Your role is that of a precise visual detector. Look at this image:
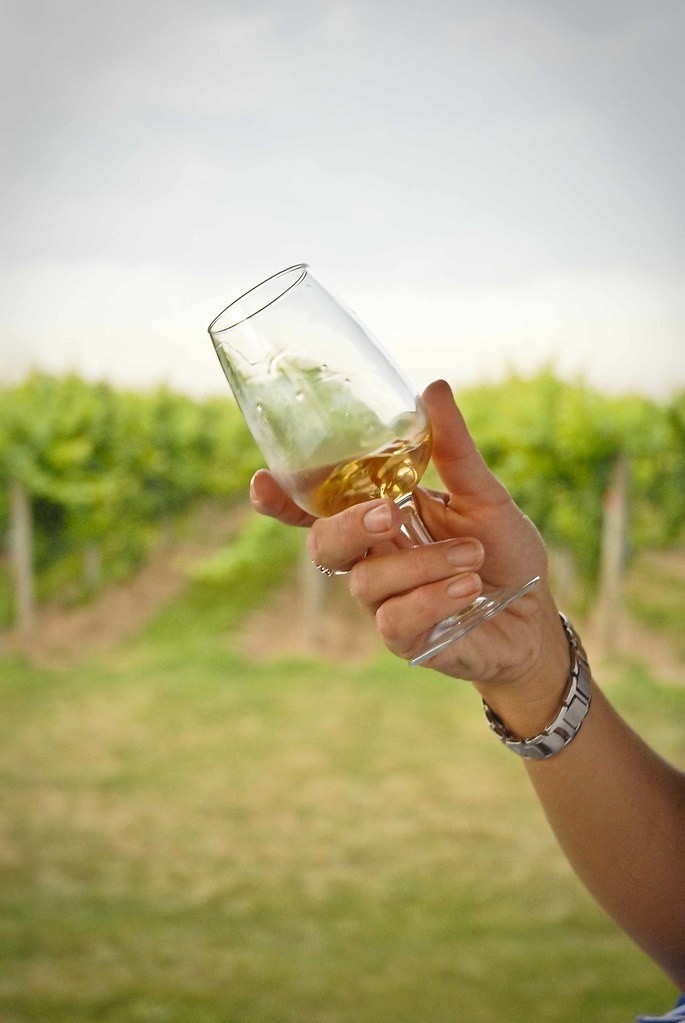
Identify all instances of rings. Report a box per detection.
[309,547,367,578]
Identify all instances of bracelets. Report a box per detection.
[481,609,593,762]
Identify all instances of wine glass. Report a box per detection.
[208,264,540,668]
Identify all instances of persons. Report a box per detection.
[249,377,685,1023]
